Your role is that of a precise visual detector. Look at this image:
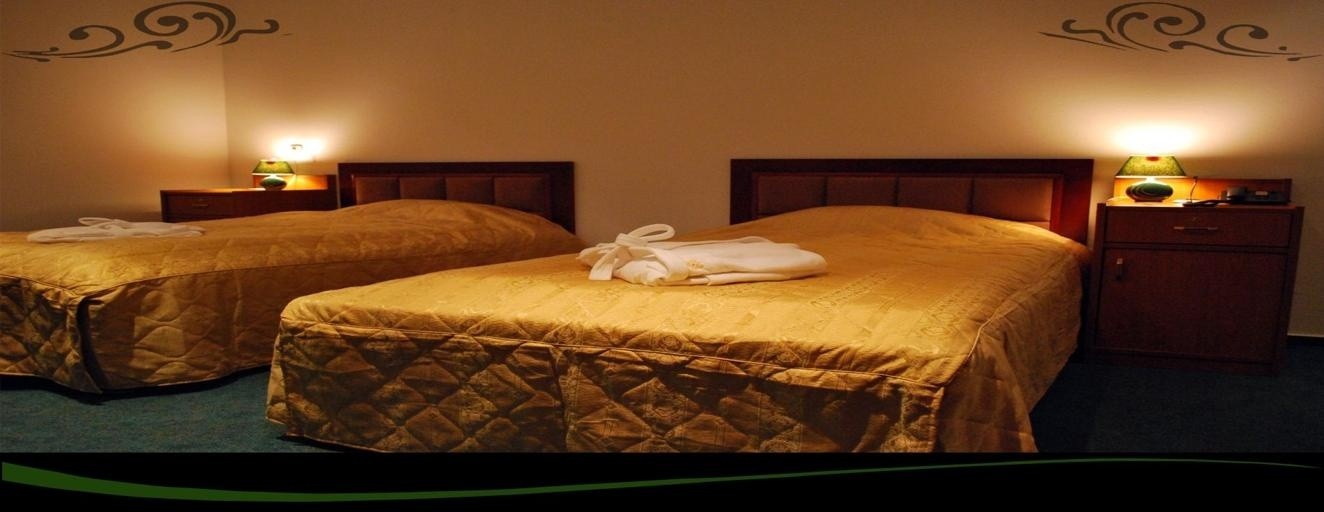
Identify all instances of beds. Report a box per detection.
[0,159,592,409]
[264,154,1095,456]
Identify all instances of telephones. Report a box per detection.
[1226,186,1287,203]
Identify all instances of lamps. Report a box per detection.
[1113,155,1187,202]
[251,159,295,190]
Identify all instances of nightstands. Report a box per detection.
[1082,173,1305,371]
[158,171,338,223]
